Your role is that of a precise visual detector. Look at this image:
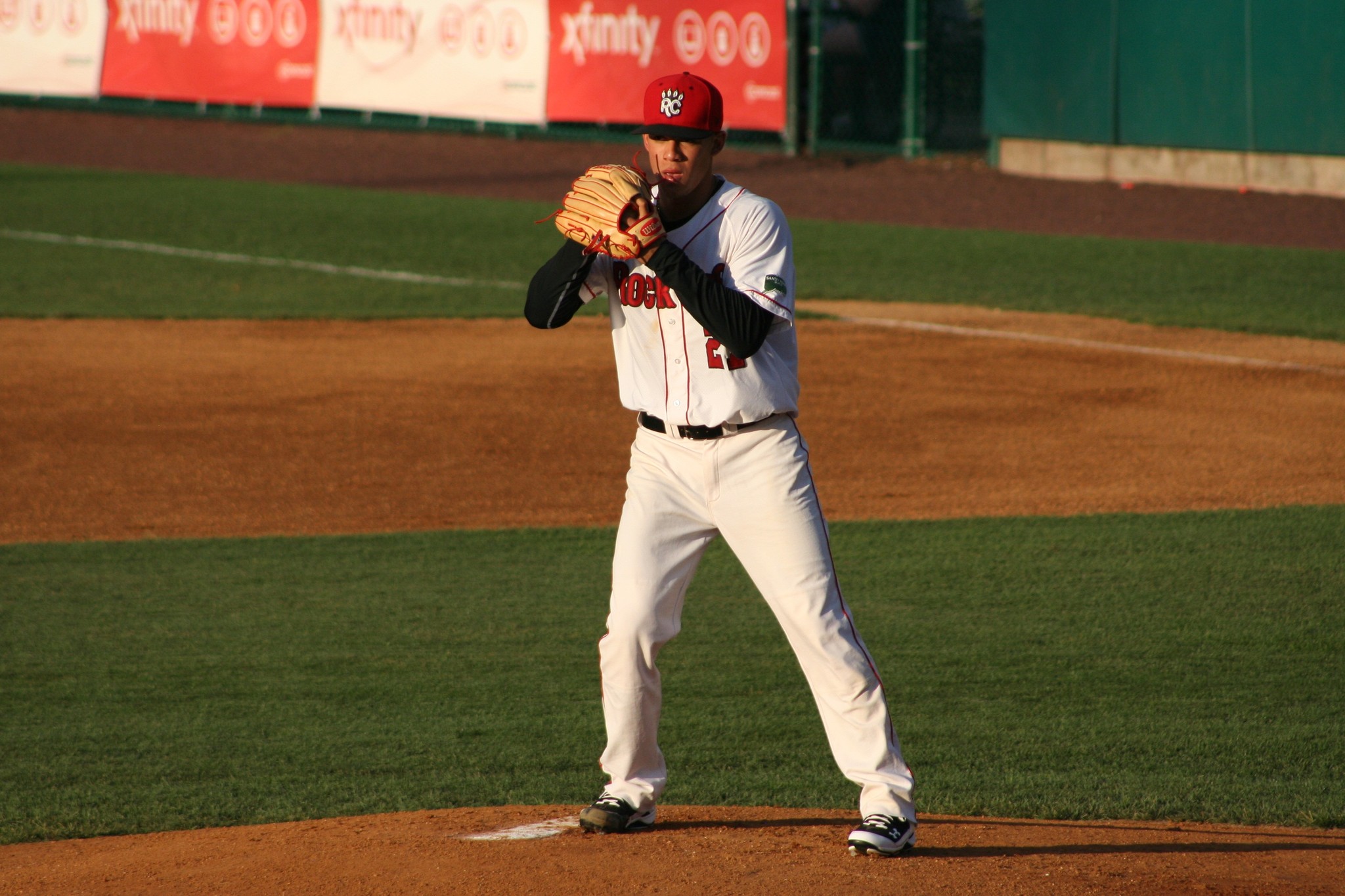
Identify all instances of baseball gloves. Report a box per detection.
[552,165,668,261]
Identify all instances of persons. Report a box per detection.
[519,71,919,860]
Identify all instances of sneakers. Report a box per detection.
[847,814,916,853]
[578,791,658,833]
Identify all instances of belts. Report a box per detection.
[640,411,774,440]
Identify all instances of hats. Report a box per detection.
[630,72,724,136]
